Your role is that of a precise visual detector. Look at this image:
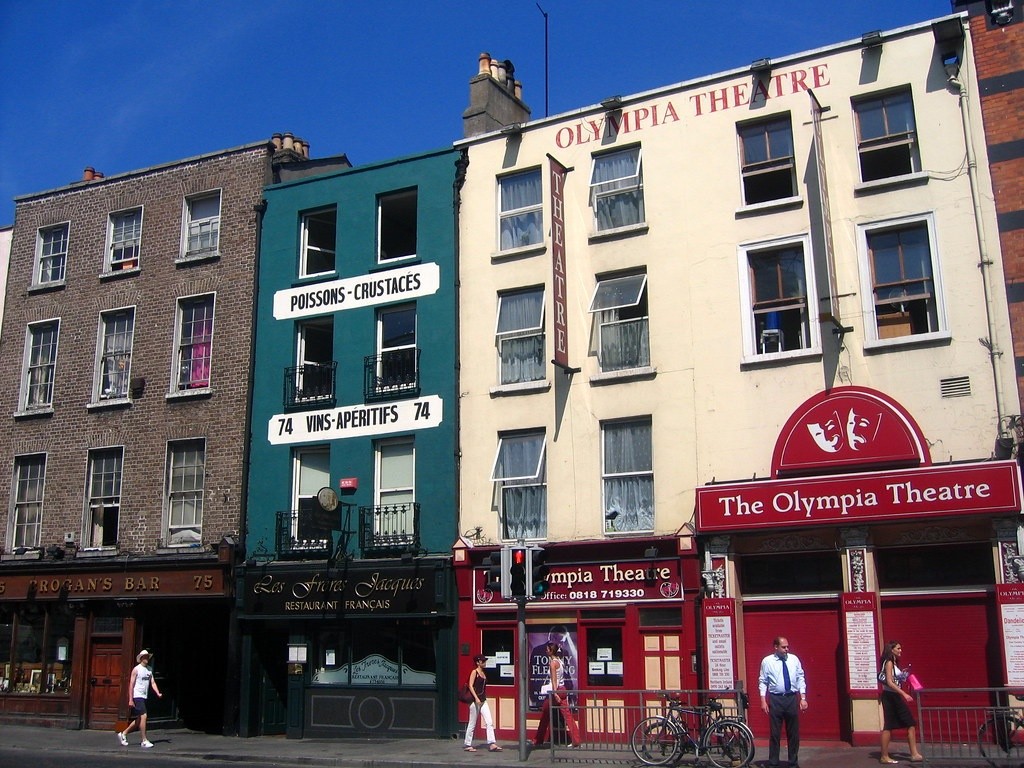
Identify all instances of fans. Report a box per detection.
[889,287,909,313]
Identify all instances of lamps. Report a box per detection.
[14,547,45,557]
[645,545,658,580]
[861,30,883,48]
[246,553,275,566]
[501,122,522,135]
[601,95,624,111]
[401,548,428,564]
[46,546,65,559]
[750,57,772,75]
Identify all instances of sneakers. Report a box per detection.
[118,732,129,746]
[140,741,153,748]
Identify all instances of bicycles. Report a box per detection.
[631,689,756,768]
[977,693,1024,768]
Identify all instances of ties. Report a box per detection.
[782,658,791,691]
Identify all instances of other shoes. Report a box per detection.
[880,758,899,764]
[912,756,929,762]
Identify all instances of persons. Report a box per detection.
[879,641,928,763]
[532,644,582,748]
[758,636,808,768]
[463,654,503,751]
[529,625,574,708]
[117,650,162,748]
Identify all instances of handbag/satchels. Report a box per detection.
[540,685,552,694]
[460,670,477,703]
[879,659,899,687]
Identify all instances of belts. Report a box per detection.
[775,693,795,696]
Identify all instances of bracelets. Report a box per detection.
[801,698,807,700]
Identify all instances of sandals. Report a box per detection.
[488,745,503,750]
[463,746,477,752]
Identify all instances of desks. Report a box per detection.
[877,311,914,338]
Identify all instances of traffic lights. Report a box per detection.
[529,548,551,599]
[508,547,530,599]
[489,548,506,599]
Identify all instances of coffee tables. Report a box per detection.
[762,329,782,353]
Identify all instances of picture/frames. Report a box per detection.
[29,669,50,692]
[4,662,10,680]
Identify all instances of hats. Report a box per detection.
[136,649,152,663]
[474,654,488,663]
